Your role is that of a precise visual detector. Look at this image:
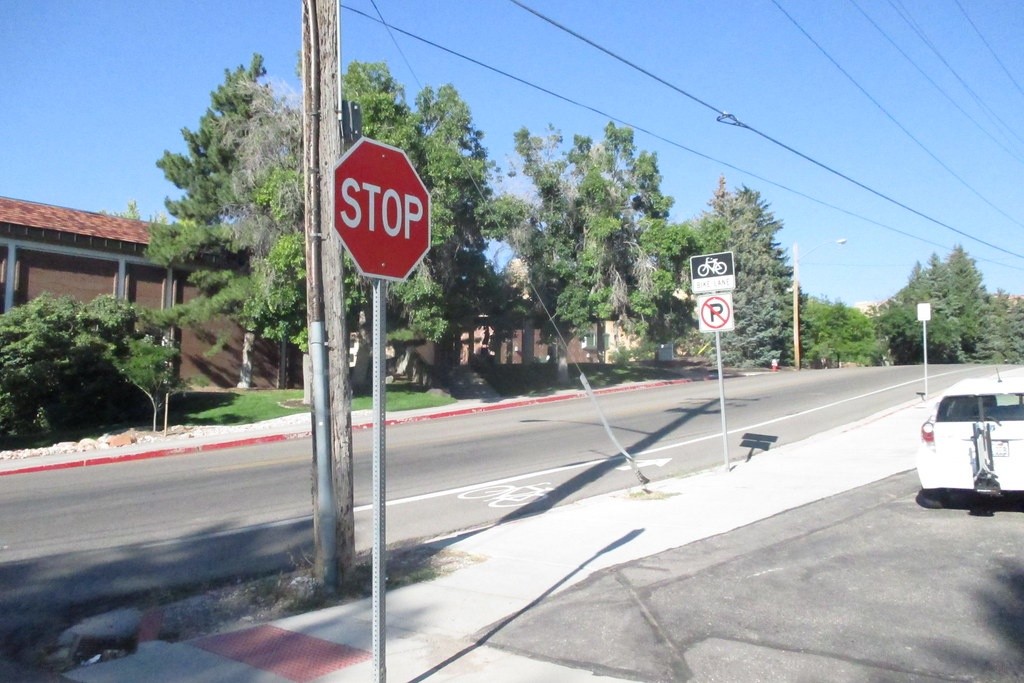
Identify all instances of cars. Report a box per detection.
[917,371,1024,495]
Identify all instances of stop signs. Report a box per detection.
[331,136,432,282]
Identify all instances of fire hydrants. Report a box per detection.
[771,358,779,372]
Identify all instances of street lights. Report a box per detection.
[793,237,846,372]
